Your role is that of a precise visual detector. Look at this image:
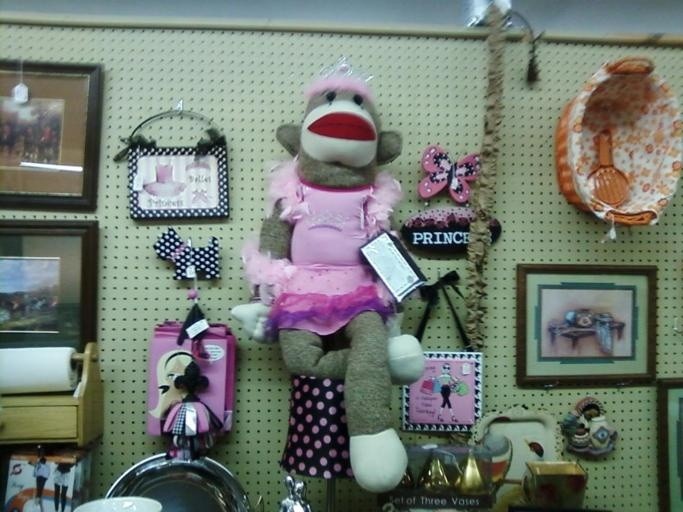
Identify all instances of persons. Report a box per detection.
[430,361,459,421]
[1,102,62,165]
[28,457,51,512]
[53,461,75,512]
[149,349,201,422]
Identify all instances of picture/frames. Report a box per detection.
[515,262,658,391]
[654,376,683,512]
[0,59,102,354]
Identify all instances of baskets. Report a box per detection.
[556,58,683,224]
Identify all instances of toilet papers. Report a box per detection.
[0,347,76,395]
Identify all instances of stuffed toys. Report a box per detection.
[230,68,426,493]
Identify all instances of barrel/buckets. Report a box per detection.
[106,455,250,510]
